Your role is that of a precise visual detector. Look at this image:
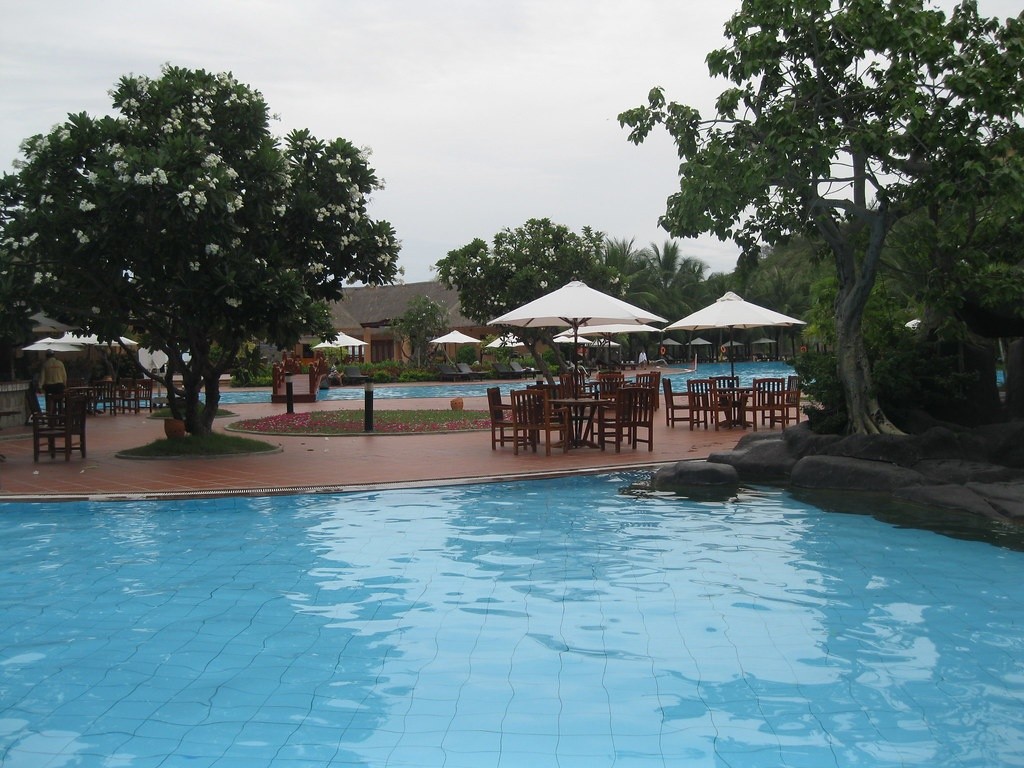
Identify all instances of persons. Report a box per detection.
[639,349,647,370]
[331,361,345,386]
[510,351,519,359]
[38,349,66,425]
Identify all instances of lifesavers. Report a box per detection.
[721,345,726,353]
[661,347,665,354]
[800,346,806,353]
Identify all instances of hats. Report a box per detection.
[45,349,54,355]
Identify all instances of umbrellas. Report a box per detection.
[685,337,712,345]
[752,337,776,353]
[313,332,368,359]
[722,341,744,347]
[485,332,531,348]
[23,326,138,359]
[551,323,665,371]
[663,292,806,388]
[429,330,481,353]
[486,281,667,398]
[656,338,683,346]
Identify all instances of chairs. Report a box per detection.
[345,366,368,385]
[597,358,639,371]
[492,362,540,380]
[438,363,487,382]
[487,371,661,456]
[663,376,801,432]
[25,378,154,463]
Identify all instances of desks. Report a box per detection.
[707,388,762,428]
[548,400,610,449]
[590,380,633,398]
